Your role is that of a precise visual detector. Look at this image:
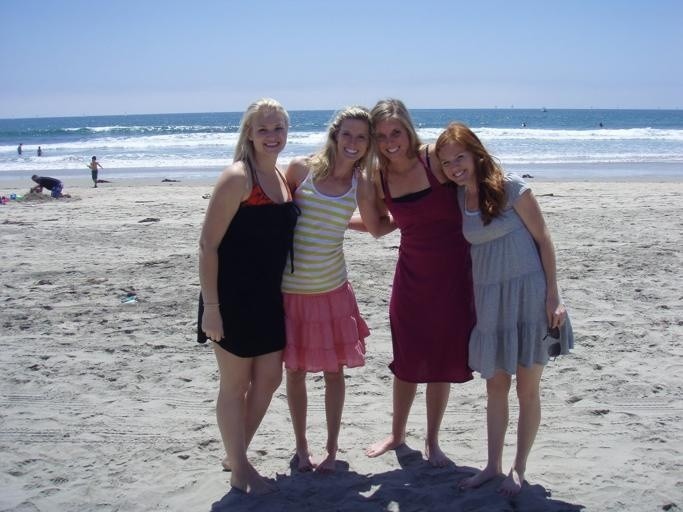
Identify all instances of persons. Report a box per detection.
[31,175,71,199]
[347,99,477,468]
[87,156,103,188]
[281,107,398,472]
[17,143,22,155]
[434,124,568,498]
[196,98,301,495]
[36,146,42,157]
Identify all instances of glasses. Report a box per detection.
[542,327,560,361]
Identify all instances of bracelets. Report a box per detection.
[203,304,219,307]
